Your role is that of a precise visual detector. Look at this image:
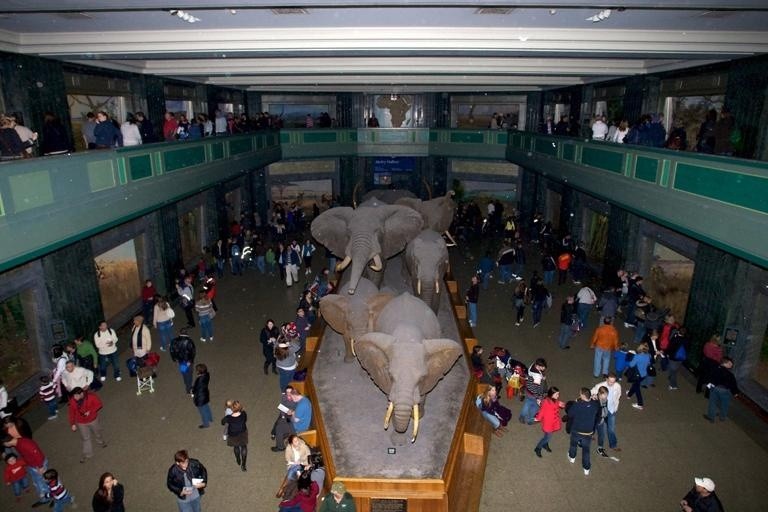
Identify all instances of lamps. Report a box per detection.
[166,7,201,25]
[585,8,612,24]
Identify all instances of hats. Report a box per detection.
[694,477,715,493]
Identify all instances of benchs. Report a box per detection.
[408,245,505,511]
[285,247,373,511]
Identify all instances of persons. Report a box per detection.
[541,109,758,162]
[304,111,332,130]
[1,111,39,161]
[368,113,380,128]
[42,112,71,156]
[81,110,282,151]
[490,112,519,129]
[0,190,740,512]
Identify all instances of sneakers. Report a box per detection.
[626,383,728,423]
[535,440,622,476]
[48,372,123,465]
[271,446,285,452]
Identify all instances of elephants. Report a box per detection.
[319,277,395,363]
[401,228,450,317]
[354,290,463,443]
[393,190,458,247]
[311,196,424,295]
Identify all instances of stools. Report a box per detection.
[135,366,153,395]
